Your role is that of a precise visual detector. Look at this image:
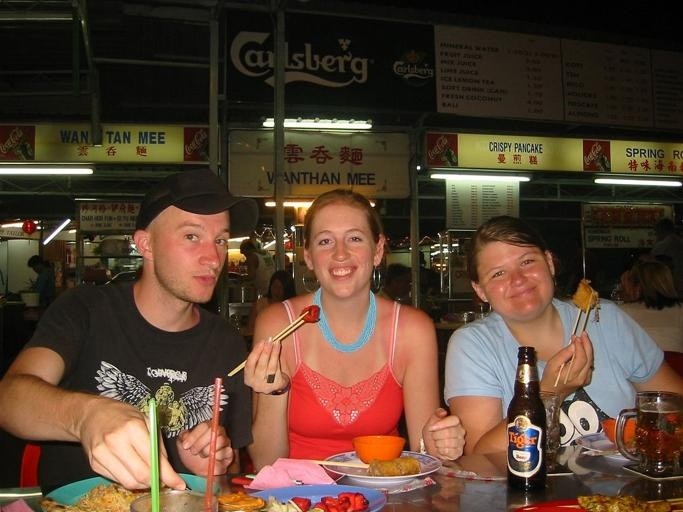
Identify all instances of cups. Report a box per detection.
[538,391,561,472]
[614,390,683,476]
[129,489,218,511]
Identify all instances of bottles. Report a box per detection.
[507,347,547,491]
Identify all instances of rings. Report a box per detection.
[267,374,276,383]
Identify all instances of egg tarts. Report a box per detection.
[218,493,265,511]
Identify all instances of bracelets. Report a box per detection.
[263,373,291,395]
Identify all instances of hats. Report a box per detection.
[136,170,259,238]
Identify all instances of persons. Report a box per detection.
[2,170,254,491]
[28,254,60,304]
[238,241,276,295]
[442,216,681,454]
[650,219,679,264]
[243,189,466,480]
[618,259,681,311]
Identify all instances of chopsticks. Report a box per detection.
[552,290,594,388]
[226,308,311,379]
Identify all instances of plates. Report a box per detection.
[321,450,442,490]
[247,484,387,512]
[575,432,637,458]
[44,472,222,512]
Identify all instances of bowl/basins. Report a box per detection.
[351,435,406,464]
[600,417,636,445]
